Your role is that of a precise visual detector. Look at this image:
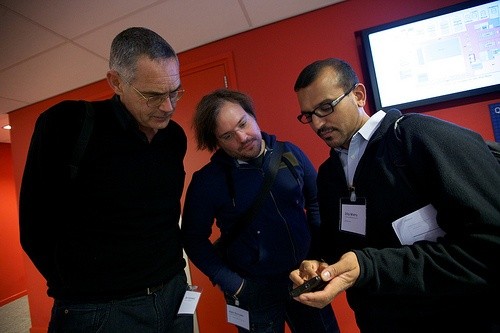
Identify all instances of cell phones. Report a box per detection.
[291,275,328,297]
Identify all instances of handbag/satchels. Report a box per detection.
[209,236,233,287]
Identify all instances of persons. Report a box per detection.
[290,58,500,333]
[19,27,194,333]
[181,89,341,333]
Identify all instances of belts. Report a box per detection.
[119,257,187,301]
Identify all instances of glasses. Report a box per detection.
[118,73,185,109]
[296,82,359,125]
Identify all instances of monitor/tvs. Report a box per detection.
[359,0,500,111]
